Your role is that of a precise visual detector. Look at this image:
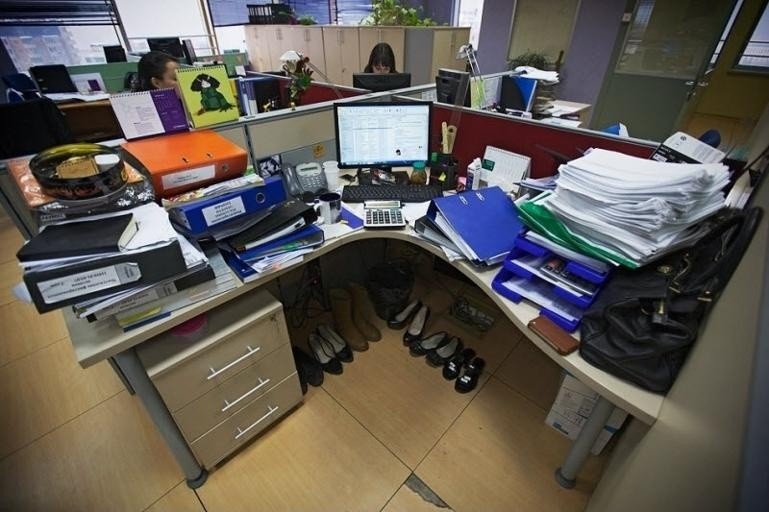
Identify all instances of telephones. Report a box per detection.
[281,161,329,197]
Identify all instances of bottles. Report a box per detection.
[323,161,340,189]
[411,163,427,186]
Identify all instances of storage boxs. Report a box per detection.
[544,368,630,456]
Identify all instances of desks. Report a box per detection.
[63,166,669,489]
[57,91,113,110]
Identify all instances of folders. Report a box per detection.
[120,128,248,202]
[169,176,288,234]
[226,197,317,254]
[510,75,539,114]
[413,186,530,267]
[235,224,324,264]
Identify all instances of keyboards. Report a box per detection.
[342,184,444,203]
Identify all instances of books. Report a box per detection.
[314,206,365,241]
[160,173,267,212]
[245,245,316,274]
[219,248,305,283]
[15,211,142,264]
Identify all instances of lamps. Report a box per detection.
[281,49,344,98]
[455,43,487,109]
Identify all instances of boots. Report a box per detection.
[329,282,380,352]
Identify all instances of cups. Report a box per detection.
[314,192,341,225]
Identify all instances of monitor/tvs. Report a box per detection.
[332,101,434,186]
[352,73,411,93]
[434,68,470,108]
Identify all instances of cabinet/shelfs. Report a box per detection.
[131,284,304,473]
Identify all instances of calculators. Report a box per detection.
[364,200,406,229]
[540,255,606,299]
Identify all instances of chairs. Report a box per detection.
[0,74,39,102]
[700,129,721,148]
[0,98,72,159]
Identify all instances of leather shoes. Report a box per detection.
[387,300,483,394]
[294,325,352,394]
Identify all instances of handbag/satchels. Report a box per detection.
[580,207,764,393]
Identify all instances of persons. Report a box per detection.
[362,42,402,75]
[130,51,181,99]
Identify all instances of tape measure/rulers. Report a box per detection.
[442,72,471,154]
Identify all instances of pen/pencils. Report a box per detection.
[404,220,419,235]
